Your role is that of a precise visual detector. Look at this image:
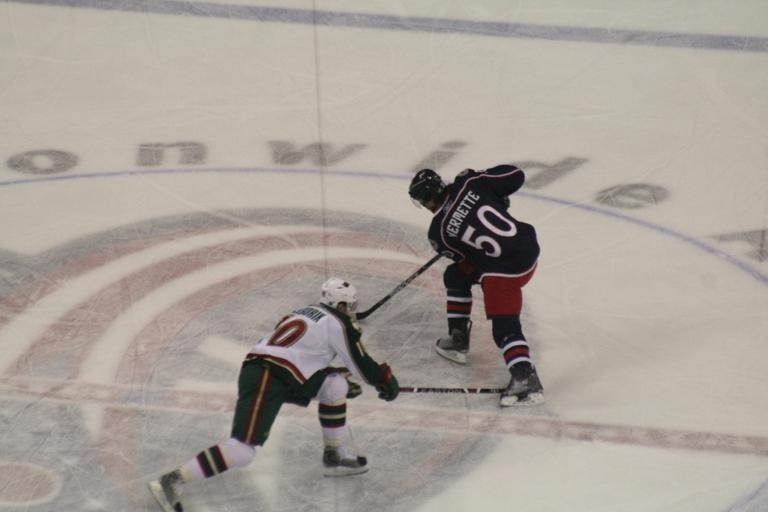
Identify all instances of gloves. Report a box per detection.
[375,361,400,401]
[338,366,362,399]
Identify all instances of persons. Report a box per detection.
[407,164,548,407]
[147,277,402,512]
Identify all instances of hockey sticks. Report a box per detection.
[355,253,442,318]
[399,374,516,393]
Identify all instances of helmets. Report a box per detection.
[317,277,358,313]
[408,168,449,214]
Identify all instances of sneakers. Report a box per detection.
[434,328,469,354]
[500,371,543,398]
[321,445,367,469]
[160,469,187,512]
